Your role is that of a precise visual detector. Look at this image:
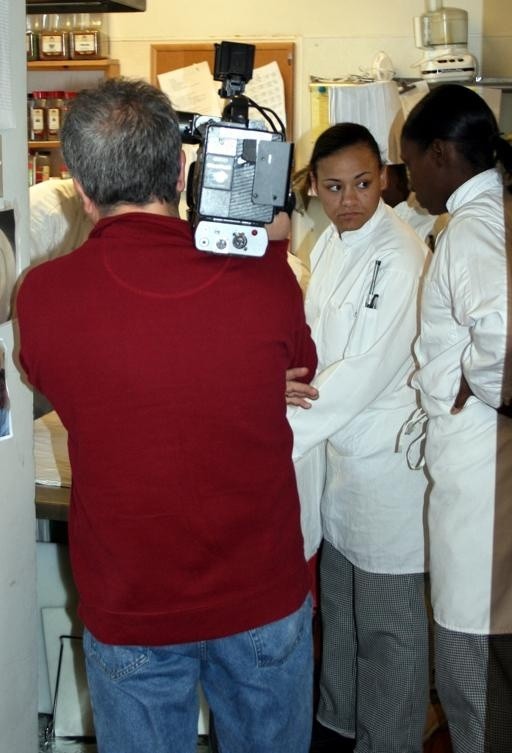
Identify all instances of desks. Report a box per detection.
[33,410,71,543]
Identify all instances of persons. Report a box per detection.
[388,76,510,751]
[9,72,326,751]
[281,119,428,751]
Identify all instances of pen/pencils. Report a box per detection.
[365,261,381,308]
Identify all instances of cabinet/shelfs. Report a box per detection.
[25,60,124,192]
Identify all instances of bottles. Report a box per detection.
[25,12,111,186]
